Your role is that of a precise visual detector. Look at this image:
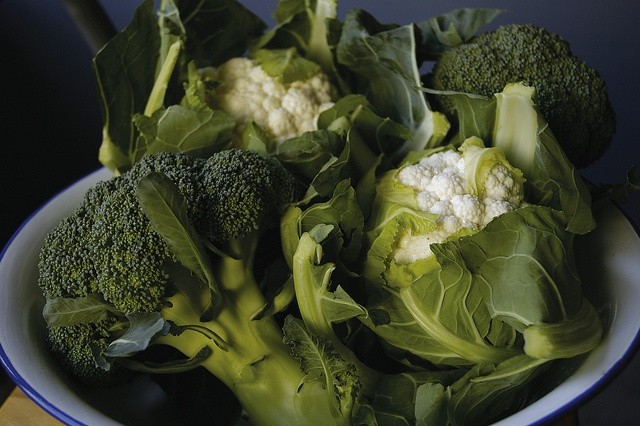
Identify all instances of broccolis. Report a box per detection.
[37,0,618,426]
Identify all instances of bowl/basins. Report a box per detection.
[2,167,638,425]
